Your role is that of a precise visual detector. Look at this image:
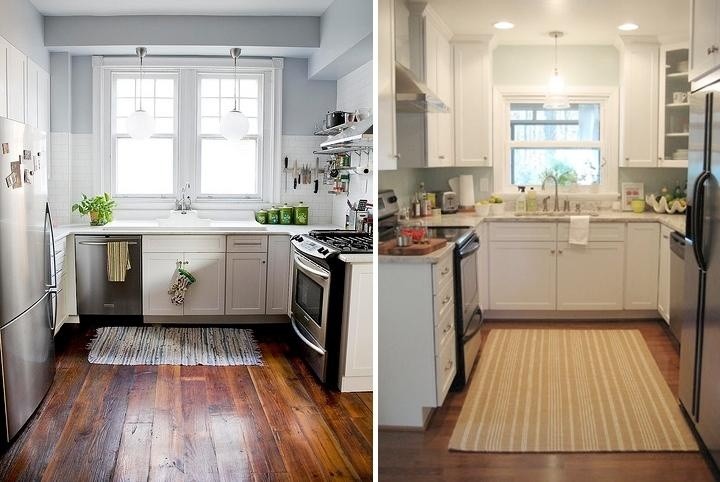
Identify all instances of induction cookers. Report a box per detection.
[378,227,475,248]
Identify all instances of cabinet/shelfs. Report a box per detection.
[658,224,677,326]
[485,220,626,311]
[379,239,458,433]
[227,235,269,316]
[626,222,662,311]
[52,237,68,335]
[687,0,720,82]
[617,34,657,167]
[269,234,292,315]
[377,1,403,171]
[311,122,359,173]
[418,5,457,168]
[142,236,228,316]
[453,40,494,168]
[658,42,691,168]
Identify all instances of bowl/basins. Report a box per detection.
[673,150,688,160]
[475,205,489,216]
[490,203,505,215]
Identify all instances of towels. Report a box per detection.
[568,215,593,246]
[105,240,132,284]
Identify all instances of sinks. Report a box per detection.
[513,211,594,221]
[158,218,211,228]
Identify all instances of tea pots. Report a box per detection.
[393,220,426,239]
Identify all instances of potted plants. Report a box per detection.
[68,190,118,227]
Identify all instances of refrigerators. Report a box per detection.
[2,116,60,447]
[676,92,720,474]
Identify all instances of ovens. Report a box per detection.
[453,233,484,390]
[288,251,345,387]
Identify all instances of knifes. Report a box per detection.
[292,156,319,194]
[285,156,289,193]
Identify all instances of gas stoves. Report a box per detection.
[289,231,373,268]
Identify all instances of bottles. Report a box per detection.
[256,201,309,225]
[674,179,687,198]
[411,183,432,217]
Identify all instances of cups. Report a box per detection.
[631,199,646,213]
[673,92,687,103]
[397,235,412,247]
[344,113,355,124]
[397,207,410,223]
[356,107,370,124]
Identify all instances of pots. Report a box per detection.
[326,111,353,129]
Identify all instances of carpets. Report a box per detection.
[446,324,705,456]
[84,323,267,370]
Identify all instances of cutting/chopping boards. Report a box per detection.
[379,238,447,256]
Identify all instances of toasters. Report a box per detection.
[434,191,459,212]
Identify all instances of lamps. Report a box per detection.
[127,47,156,144]
[540,30,572,111]
[219,48,250,142]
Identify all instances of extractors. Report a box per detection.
[320,119,373,148]
[396,1,453,113]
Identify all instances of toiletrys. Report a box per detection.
[515,185,527,213]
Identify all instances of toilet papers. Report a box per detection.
[448,173,475,207]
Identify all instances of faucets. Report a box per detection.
[541,174,559,212]
[180,183,191,215]
